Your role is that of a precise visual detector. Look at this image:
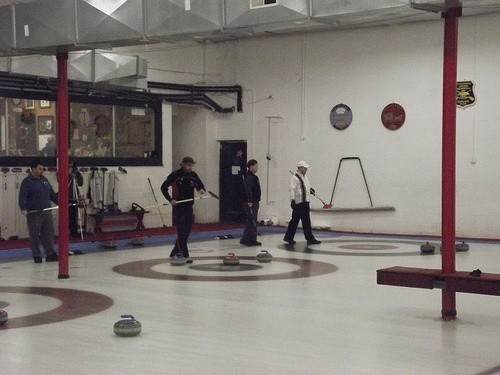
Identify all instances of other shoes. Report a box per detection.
[46,255,58,261]
[283,237,296,244]
[239,240,261,246]
[307,239,321,244]
[185,257,193,263]
[34,256,42,263]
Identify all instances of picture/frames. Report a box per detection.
[24,99,35,110]
[37,114,55,135]
[39,100,51,108]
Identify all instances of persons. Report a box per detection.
[56,158,84,237]
[161,157,206,263]
[41,136,120,158]
[239,159,262,246]
[283,161,322,244]
[18,161,59,263]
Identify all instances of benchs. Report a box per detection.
[376,265,500,297]
[89,210,150,233]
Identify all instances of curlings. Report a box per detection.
[131,235,145,245]
[421,242,435,252]
[170,253,186,266]
[257,250,272,263]
[103,239,117,249]
[0,309,8,326]
[114,314,142,337]
[455,241,469,251]
[223,253,240,265]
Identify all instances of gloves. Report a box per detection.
[291,201,296,209]
[310,188,315,194]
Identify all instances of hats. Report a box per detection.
[180,157,197,166]
[298,161,309,168]
